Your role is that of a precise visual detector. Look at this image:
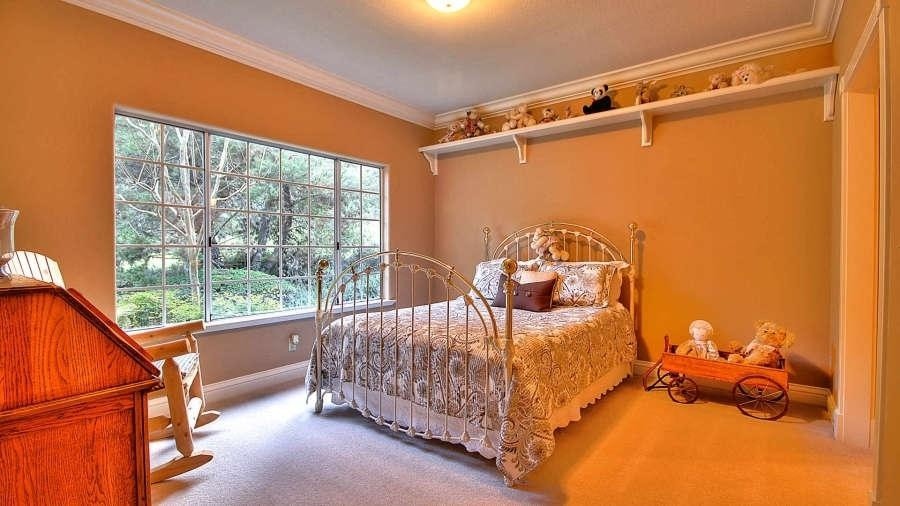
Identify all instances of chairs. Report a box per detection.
[126,318,218,485]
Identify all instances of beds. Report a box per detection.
[305,222,639,489]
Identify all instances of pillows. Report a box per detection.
[466,259,630,312]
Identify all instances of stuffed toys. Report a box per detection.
[440,107,490,145]
[675,319,720,361]
[443,63,762,144]
[727,318,795,367]
[583,84,616,117]
[671,85,692,97]
[533,227,569,260]
[703,62,762,94]
[632,79,656,106]
[501,104,573,132]
